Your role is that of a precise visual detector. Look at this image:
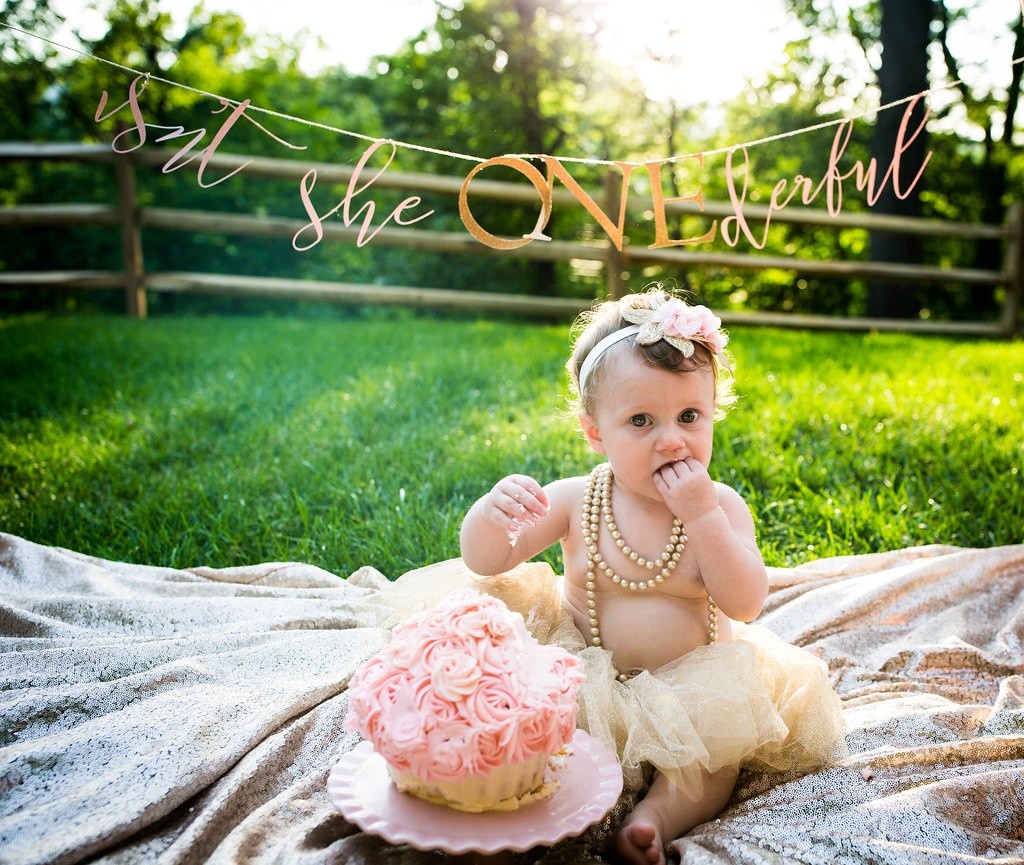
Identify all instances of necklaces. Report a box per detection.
[581,461,716,685]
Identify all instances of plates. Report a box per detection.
[323,727,625,859]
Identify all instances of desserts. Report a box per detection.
[344,588,586,812]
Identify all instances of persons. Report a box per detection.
[458,280,848,865]
